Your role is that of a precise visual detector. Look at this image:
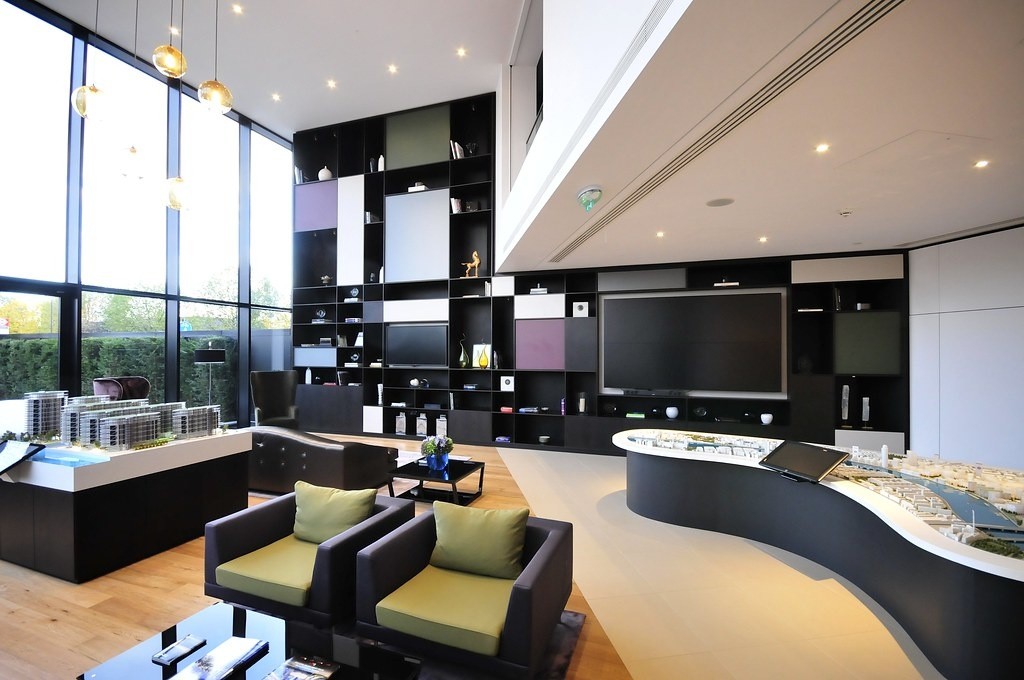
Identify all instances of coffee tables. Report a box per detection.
[76,602,422,680]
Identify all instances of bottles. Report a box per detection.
[459,346,469,368]
[479,348,488,369]
[317,166,332,181]
[305,367,311,384]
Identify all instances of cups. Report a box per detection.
[451,197,462,214]
[666,407,678,419]
[760,413,773,424]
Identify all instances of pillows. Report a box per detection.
[294,480,378,544]
[429,501,530,579]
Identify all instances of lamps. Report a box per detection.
[198,0,233,116]
[161,0,193,210]
[152,0,187,78]
[71,0,107,119]
[577,187,603,212]
[195,341,225,406]
[119,0,149,180]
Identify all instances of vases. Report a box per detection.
[427,452,448,470]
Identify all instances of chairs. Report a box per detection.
[250,370,299,431]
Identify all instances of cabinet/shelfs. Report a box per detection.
[292,92,910,457]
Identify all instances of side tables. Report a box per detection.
[385,457,485,506]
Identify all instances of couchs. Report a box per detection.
[205,492,415,630]
[356,505,573,680]
[239,426,398,496]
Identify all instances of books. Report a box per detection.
[450,140,464,159]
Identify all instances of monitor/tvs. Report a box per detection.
[759,440,849,484]
[598,285,793,401]
[0,440,46,476]
[385,325,448,368]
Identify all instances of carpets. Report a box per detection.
[248,449,472,499]
[213,599,586,680]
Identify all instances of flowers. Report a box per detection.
[421,435,453,465]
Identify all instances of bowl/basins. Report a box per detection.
[539,436,550,443]
